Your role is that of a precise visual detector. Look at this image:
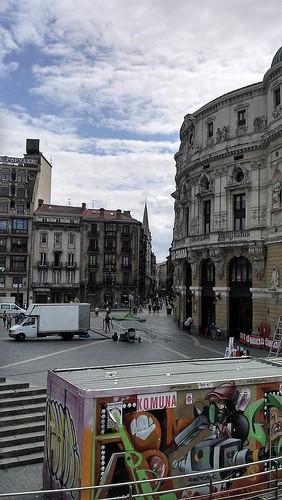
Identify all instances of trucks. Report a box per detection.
[8,303,91,342]
[42,355,282,500]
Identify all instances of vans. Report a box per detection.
[0,303,27,320]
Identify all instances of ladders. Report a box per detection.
[267,316,282,356]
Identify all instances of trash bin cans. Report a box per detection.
[167,308,171,315]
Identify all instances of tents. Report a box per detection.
[111,312,146,341]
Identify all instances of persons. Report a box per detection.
[2,295,221,341]
